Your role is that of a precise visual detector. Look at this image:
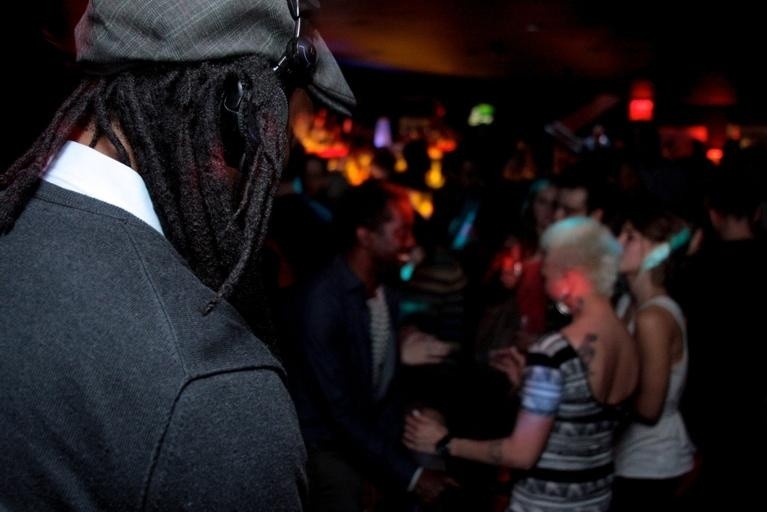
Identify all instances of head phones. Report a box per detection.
[224,0,318,167]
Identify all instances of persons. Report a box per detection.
[1,0,360,511]
[199,124,765,512]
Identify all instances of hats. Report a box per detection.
[72,0,360,120]
